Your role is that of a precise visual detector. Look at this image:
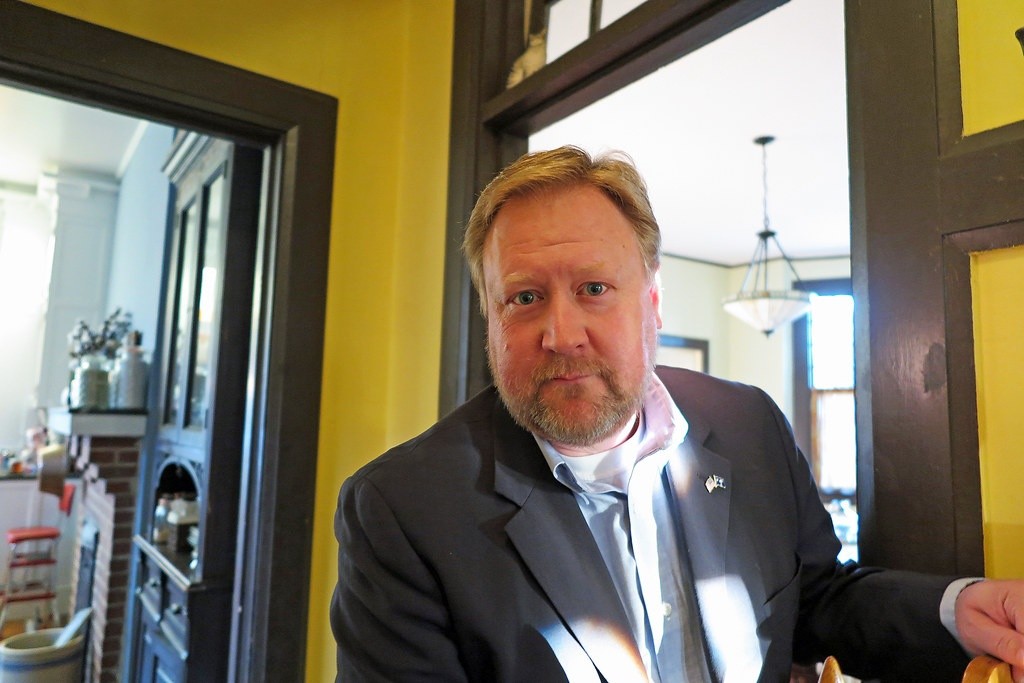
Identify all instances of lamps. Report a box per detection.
[719,136,819,340]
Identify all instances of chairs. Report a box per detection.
[0,482,77,644]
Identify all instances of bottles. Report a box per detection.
[118,346,147,408]
[152,498,168,544]
[167,493,197,553]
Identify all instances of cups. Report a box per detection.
[0,628,84,683]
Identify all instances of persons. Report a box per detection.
[330,146,1024,683]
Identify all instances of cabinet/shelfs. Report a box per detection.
[115,122,267,683]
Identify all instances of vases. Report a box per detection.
[81,350,110,375]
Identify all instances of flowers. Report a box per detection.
[67,307,132,358]
